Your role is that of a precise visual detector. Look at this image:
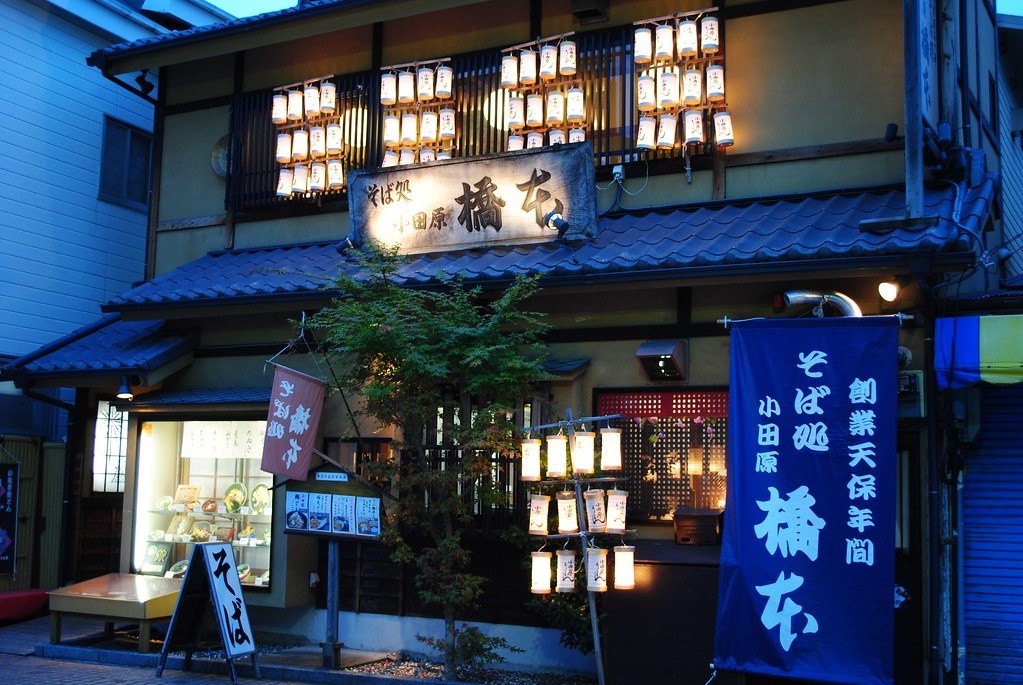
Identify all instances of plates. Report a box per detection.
[224,482,248,511]
[250,484,271,512]
[156,496,173,509]
[285,510,378,535]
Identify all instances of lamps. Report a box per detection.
[335,233,358,257]
[134,69,154,95]
[634,338,690,384]
[885,124,905,142]
[116,376,133,398]
[545,212,569,243]
[570,0,610,25]
[878,273,913,301]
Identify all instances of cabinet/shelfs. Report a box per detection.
[146,510,272,587]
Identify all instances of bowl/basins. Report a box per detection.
[171,558,190,576]
[193,520,211,535]
[236,564,252,580]
[202,499,217,513]
